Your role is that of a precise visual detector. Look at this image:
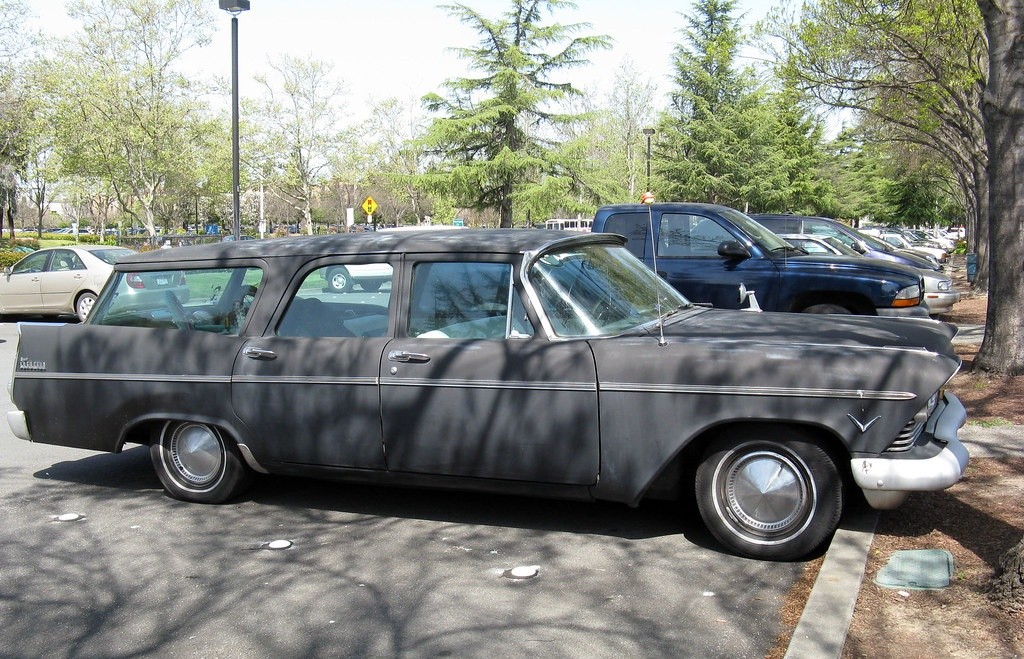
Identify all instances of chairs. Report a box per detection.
[58,256,75,270]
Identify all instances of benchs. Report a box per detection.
[415,315,526,338]
[340,312,388,336]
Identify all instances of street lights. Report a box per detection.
[642,129,655,192]
[218,0,250,241]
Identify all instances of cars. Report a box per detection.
[222,236,255,242]
[3,223,972,562]
[20,228,163,236]
[318,261,404,294]
[689,208,966,317]
[0,245,189,321]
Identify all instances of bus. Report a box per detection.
[544,219,593,235]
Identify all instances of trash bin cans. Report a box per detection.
[966,252,977,282]
[12,246,35,253]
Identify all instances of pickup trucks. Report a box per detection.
[439,201,933,333]
